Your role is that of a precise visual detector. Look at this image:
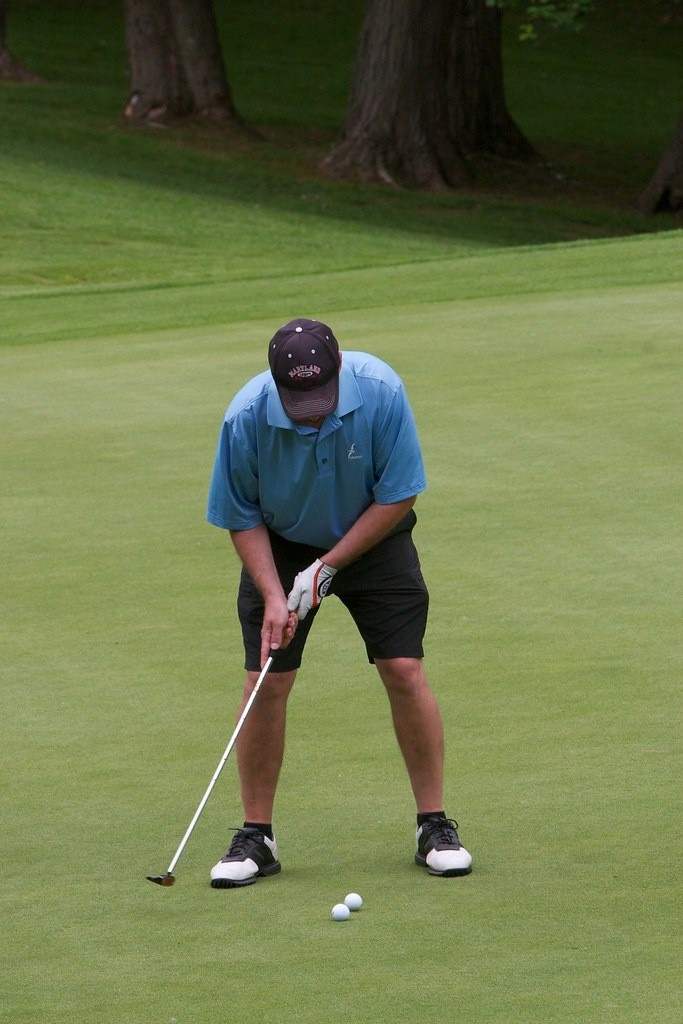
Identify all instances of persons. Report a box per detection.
[204,319,473,890]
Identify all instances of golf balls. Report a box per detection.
[344,893,363,911]
[331,903,351,921]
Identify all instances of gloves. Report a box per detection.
[286,558,337,620]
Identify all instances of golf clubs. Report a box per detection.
[145,608,298,888]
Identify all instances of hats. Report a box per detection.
[267,318,341,419]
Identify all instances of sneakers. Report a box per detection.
[210,828,282,889]
[415,816,472,877]
